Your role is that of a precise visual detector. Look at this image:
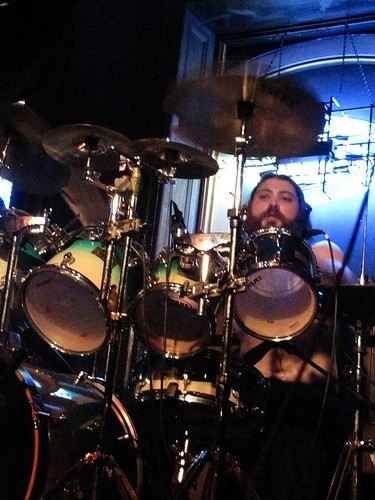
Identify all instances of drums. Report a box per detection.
[229,227,322,344]
[0,360,145,500]
[131,348,272,441]
[127,245,224,359]
[0,207,73,310]
[20,233,152,357]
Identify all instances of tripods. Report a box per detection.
[1,122,374,500]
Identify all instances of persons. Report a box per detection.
[222,171,356,500]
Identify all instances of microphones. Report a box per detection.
[295,230,322,241]
[170,201,190,238]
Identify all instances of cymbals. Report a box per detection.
[161,70,327,159]
[113,137,220,180]
[0,101,71,196]
[40,123,133,173]
